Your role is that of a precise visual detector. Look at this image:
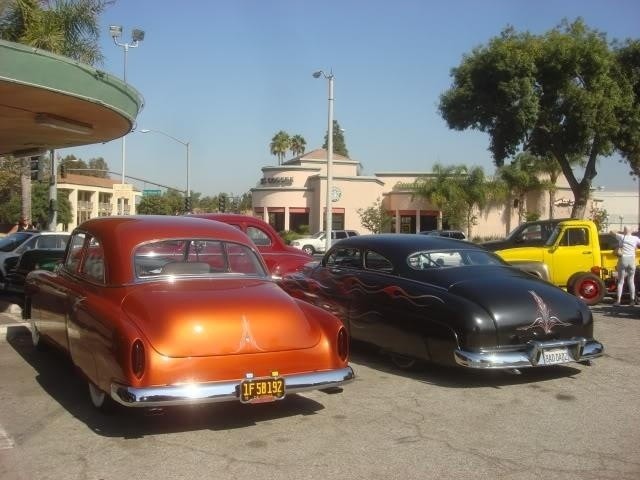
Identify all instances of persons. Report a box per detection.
[8,215,38,234]
[609,225,640,310]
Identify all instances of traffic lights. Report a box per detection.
[218,195,226,213]
[59,164,67,179]
[183,195,193,211]
[30,155,41,181]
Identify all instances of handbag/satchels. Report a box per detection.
[613,247,623,256]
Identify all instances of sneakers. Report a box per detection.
[629,300,636,307]
[614,302,622,307]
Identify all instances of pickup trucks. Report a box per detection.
[471,216,640,299]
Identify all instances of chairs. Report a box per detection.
[161,263,209,278]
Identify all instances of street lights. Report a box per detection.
[139,128,194,215]
[311,67,339,256]
[107,24,147,221]
